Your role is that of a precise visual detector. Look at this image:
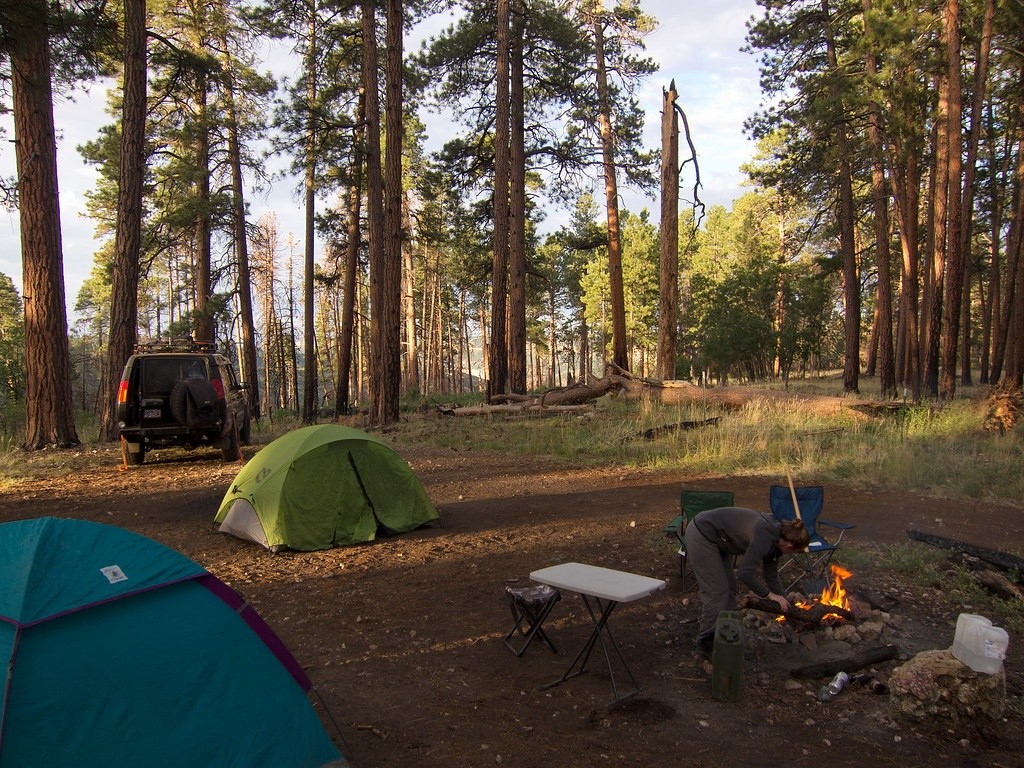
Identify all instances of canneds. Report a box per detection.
[828,671,848,694]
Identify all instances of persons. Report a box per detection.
[940,368,953,403]
[903,384,909,403]
[684,506,810,660]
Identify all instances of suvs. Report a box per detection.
[115,336,251,464]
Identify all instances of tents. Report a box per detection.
[211,423,443,555]
[0,515,363,768]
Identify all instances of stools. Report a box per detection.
[500,583,563,656]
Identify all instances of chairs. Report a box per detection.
[663,488,736,593]
[768,485,857,591]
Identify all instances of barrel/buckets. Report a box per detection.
[712,610,745,702]
[952,612,1010,675]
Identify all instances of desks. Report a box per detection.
[527,560,666,711]
